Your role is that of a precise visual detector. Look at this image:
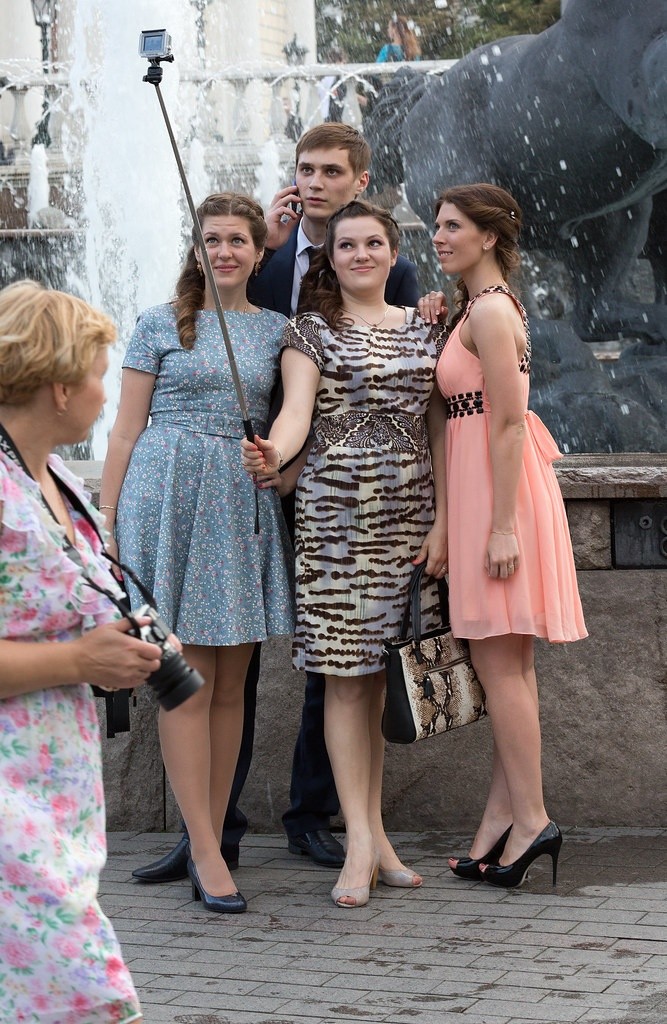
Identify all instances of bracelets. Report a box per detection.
[277,450,284,469]
[99,506,116,510]
[491,531,515,534]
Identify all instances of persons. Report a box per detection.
[97,192,308,912]
[378,16,421,63]
[419,183,588,886]
[132,124,419,883]
[325,50,375,124]
[0,280,181,1024]
[240,199,451,909]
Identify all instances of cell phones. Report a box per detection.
[292,180,297,215]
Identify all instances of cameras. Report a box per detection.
[101,604,206,713]
[138,32,172,58]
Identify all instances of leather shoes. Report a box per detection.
[130,827,239,882]
[287,829,347,868]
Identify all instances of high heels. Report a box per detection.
[187,857,247,913]
[481,820,562,888]
[378,864,423,888]
[332,858,379,907]
[449,823,530,880]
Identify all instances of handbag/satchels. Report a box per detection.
[380,562,486,743]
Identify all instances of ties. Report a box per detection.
[298,246,328,315]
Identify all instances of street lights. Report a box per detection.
[32,0,56,147]
[282,31,308,143]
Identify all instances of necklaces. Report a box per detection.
[202,302,250,311]
[340,304,390,327]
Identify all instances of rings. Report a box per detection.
[442,567,446,571]
[508,565,515,568]
